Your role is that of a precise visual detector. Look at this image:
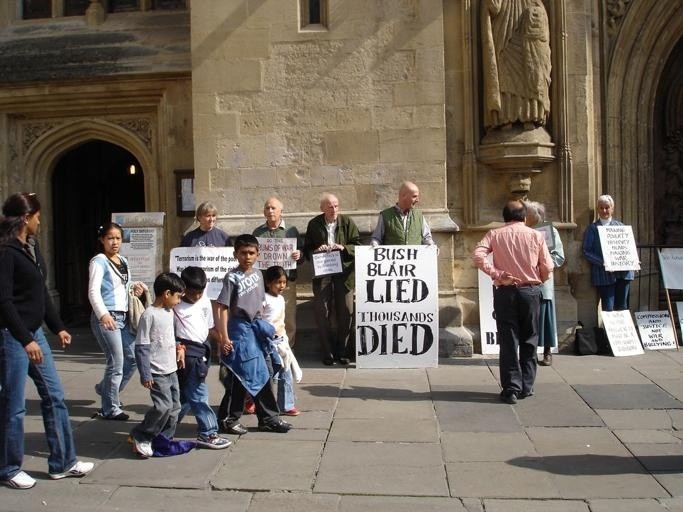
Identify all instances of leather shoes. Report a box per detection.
[322,356,351,367]
[541,352,553,366]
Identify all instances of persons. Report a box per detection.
[471,199,556,405]
[124,271,187,459]
[521,199,567,366]
[304,191,363,367]
[86,221,144,421]
[369,180,440,257]
[250,197,306,352]
[172,265,232,450]
[582,194,641,312]
[0,192,94,489]
[178,201,230,247]
[242,264,300,417]
[215,234,293,434]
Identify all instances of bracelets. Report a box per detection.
[176,344,186,350]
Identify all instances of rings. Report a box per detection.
[31,356,35,360]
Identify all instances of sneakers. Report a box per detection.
[131,432,154,456]
[4,471,36,489]
[280,407,300,415]
[198,435,232,449]
[223,419,246,434]
[95,408,129,421]
[44,461,93,480]
[499,388,518,401]
[259,416,293,433]
